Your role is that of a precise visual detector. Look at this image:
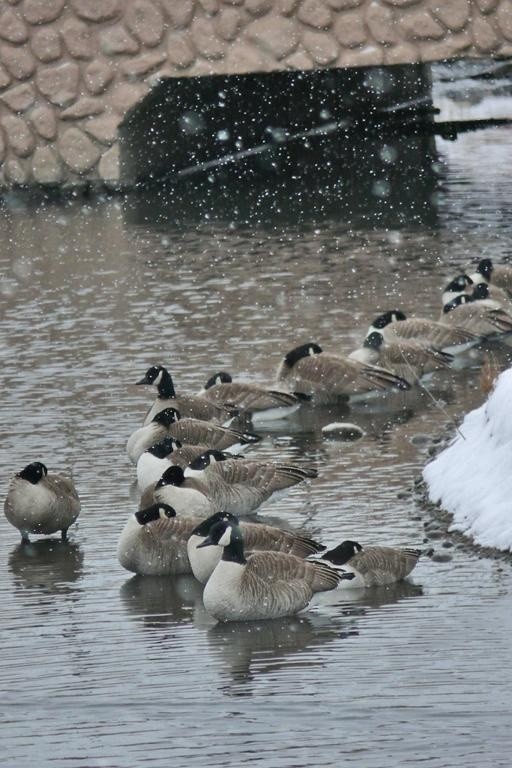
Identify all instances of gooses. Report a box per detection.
[3,461,81,544]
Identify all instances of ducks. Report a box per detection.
[118,259,511,623]
